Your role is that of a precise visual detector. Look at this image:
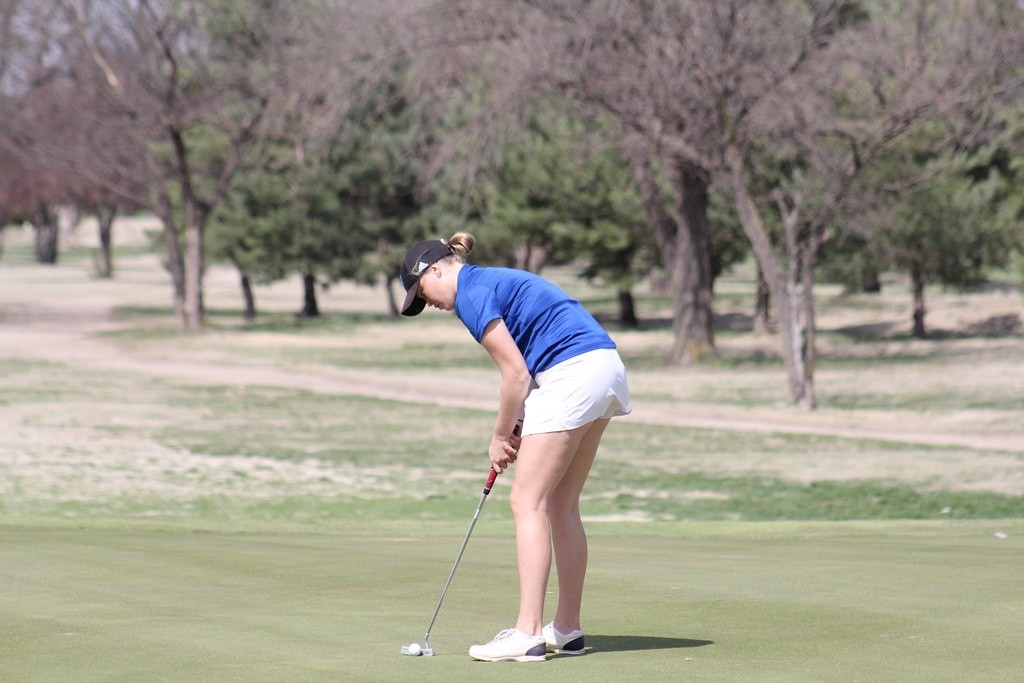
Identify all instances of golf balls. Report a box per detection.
[401,423,520,656]
[408,643,421,656]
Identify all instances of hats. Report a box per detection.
[400,240,453,318]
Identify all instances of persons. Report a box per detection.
[399,231,634,663]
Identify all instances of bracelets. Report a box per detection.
[516,417,523,423]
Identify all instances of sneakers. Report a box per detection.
[469,630,547,662]
[542,623,586,655]
